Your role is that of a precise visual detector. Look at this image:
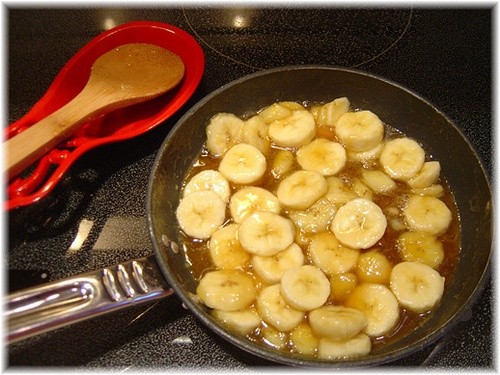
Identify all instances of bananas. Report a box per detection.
[175,96,452,359]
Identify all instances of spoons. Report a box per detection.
[3,22,204,209]
[2,42,185,183]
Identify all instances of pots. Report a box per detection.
[4,67,493,371]
